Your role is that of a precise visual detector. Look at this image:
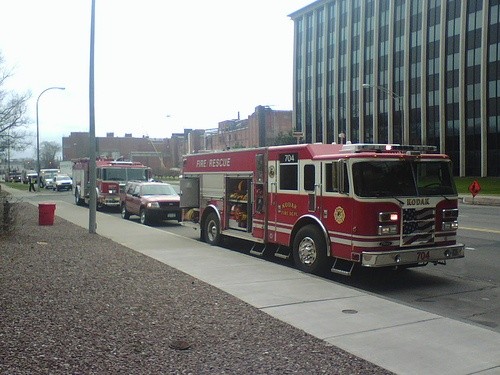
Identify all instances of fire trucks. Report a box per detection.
[179,143,465,276]
[72,157,152,211]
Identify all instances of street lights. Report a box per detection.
[363,82,405,144]
[36,86,65,173]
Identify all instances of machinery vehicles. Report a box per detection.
[5,169,21,183]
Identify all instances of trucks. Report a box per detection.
[40,169,58,188]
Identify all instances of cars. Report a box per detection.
[53,176,72,191]
[119,181,180,224]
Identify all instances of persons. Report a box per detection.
[29,176,36,192]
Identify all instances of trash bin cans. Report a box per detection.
[38,203,56,225]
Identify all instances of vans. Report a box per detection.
[22,169,39,184]
